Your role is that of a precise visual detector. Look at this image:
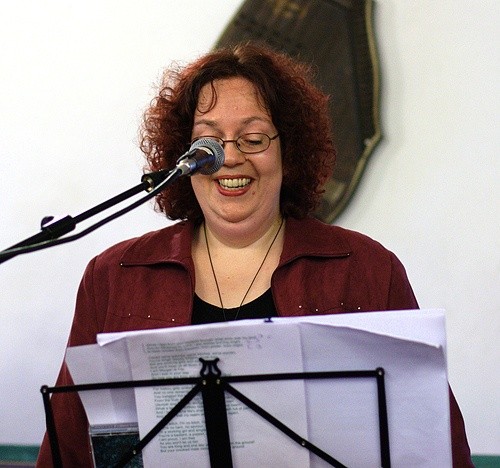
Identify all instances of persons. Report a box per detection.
[33,40,477,468]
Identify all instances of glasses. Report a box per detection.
[187,132,281,154]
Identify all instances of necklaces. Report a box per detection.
[202,218,285,321]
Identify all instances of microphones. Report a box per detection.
[175,138,225,177]
